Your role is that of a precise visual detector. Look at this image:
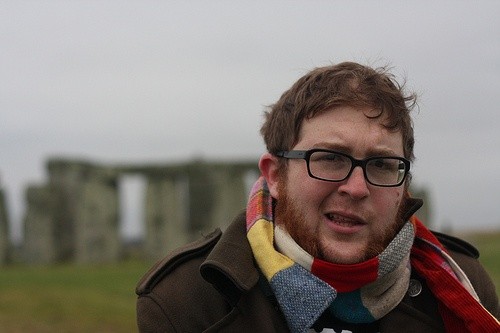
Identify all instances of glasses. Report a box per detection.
[274,148,410,187]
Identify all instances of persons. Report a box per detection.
[133,62,500,333]
